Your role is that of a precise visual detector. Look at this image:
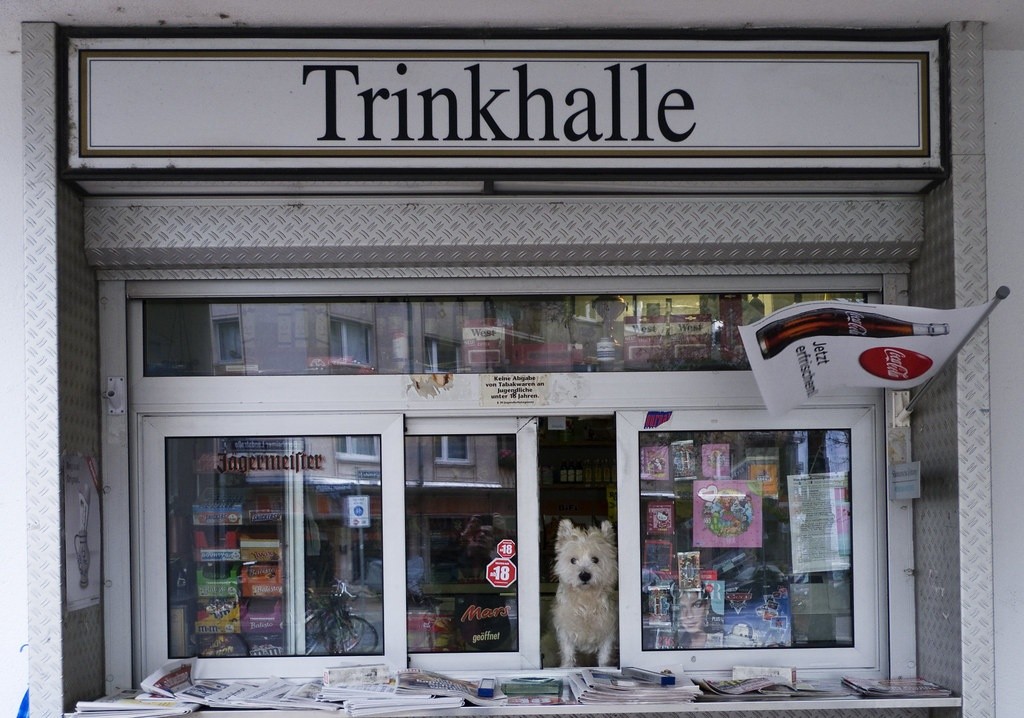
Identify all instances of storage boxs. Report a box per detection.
[639,443,792,650]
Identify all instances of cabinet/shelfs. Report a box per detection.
[537,416,617,490]
[192,486,286,656]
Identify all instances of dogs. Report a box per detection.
[550,518,618,668]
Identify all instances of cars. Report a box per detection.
[717,561,789,616]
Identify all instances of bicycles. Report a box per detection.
[279,578,380,656]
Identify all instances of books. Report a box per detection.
[841,676,951,698]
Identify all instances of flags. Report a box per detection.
[736,301,995,419]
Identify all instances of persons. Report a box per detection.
[668,579,723,649]
[437,511,512,652]
